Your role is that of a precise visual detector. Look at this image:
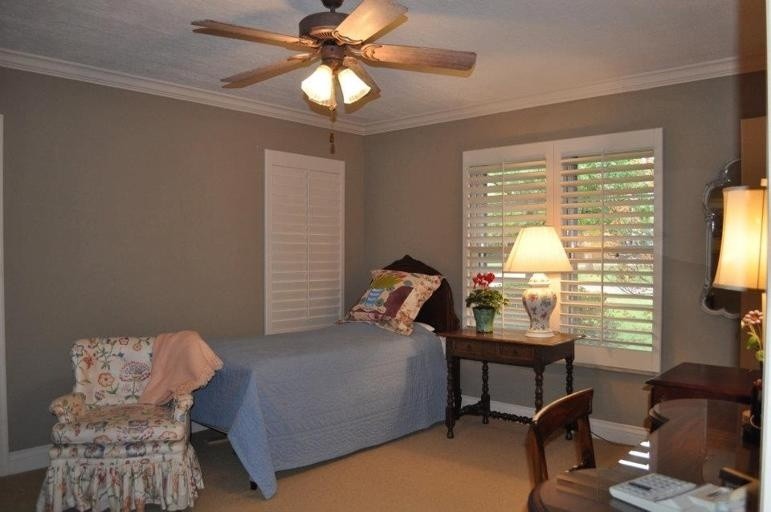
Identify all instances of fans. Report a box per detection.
[191,1,477,118]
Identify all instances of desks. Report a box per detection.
[437,328,576,440]
[528,395,763,512]
[644,362,764,475]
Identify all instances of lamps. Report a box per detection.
[301,43,378,109]
[501,227,573,338]
[712,185,768,348]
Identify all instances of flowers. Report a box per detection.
[741,309,764,415]
[465,272,509,315]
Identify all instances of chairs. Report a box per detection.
[525,388,595,487]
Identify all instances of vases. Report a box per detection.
[472,306,495,333]
[750,415,761,446]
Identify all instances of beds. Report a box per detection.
[190,255,460,490]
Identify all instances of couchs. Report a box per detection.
[36,337,204,512]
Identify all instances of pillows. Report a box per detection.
[335,269,444,335]
